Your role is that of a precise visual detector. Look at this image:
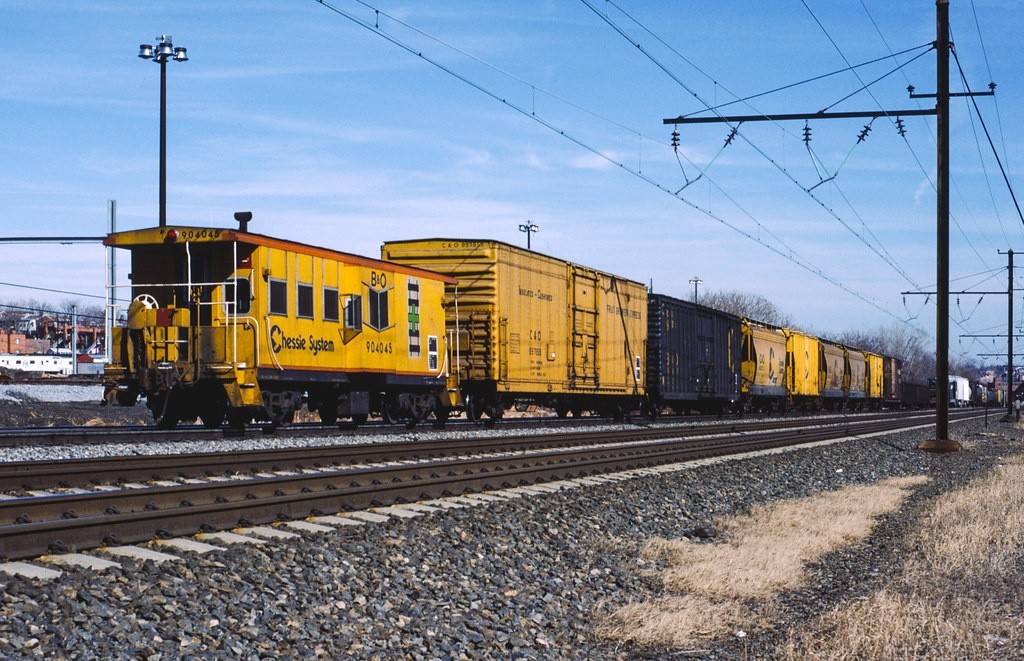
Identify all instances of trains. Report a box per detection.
[99,212,1004,428]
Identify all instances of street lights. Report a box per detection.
[136,36,189,227]
[689,276,704,302]
[519,218,539,249]
